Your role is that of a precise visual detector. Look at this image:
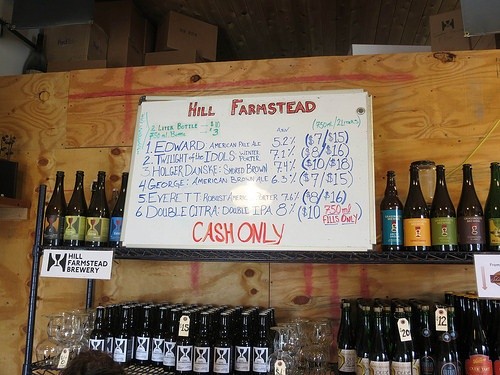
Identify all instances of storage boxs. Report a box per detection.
[43,0,217,74]
[428,9,497,51]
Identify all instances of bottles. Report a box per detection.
[108,171,130,248]
[484,161,500,251]
[63,170,88,247]
[110,188,119,216]
[380,170,404,252]
[42,170,67,246]
[430,165,458,251]
[457,164,487,251]
[89,300,274,375]
[23,33,47,74]
[336,289,500,375]
[404,164,431,252]
[85,171,110,247]
[89,181,98,211]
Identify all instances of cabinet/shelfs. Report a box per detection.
[23,183,500,375]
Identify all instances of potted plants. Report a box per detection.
[0,135,20,199]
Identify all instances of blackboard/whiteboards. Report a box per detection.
[115,87,377,253]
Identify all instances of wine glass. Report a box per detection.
[36,305,98,370]
[266,316,334,374]
